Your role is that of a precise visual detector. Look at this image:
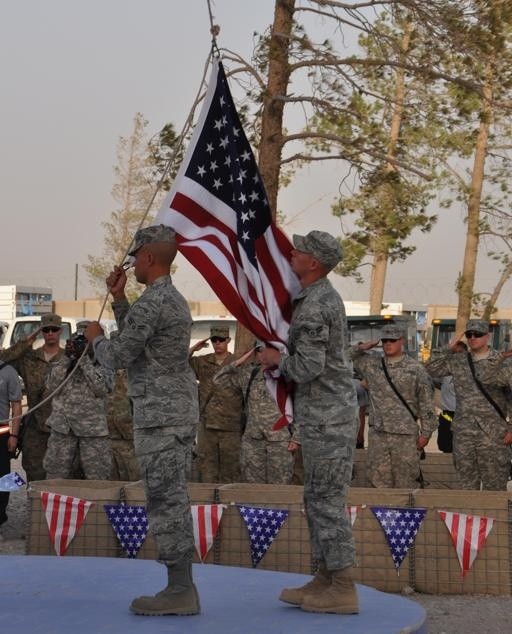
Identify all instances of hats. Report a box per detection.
[76,321,89,335]
[465,319,489,333]
[41,314,61,328]
[210,325,229,339]
[380,325,402,340]
[128,224,176,256]
[293,230,343,269]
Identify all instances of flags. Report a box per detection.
[155,55,303,431]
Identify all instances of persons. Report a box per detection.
[437,340,469,452]
[425,320,512,492]
[103,329,138,482]
[0,328,23,523]
[84,224,200,616]
[45,320,113,482]
[348,323,438,489]
[259,230,359,615]
[183,323,247,486]
[352,367,368,449]
[2,310,66,482]
[211,344,301,485]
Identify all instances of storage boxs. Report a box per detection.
[353,448,372,486]
[216,484,312,575]
[412,489,511,596]
[123,480,215,565]
[421,454,459,487]
[339,487,411,597]
[26,478,122,558]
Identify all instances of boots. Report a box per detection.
[279,559,331,605]
[129,561,202,616]
[302,565,359,614]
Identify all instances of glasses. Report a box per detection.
[465,331,485,338]
[211,337,226,342]
[381,339,399,343]
[42,327,59,333]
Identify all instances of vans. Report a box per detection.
[0,316,115,390]
